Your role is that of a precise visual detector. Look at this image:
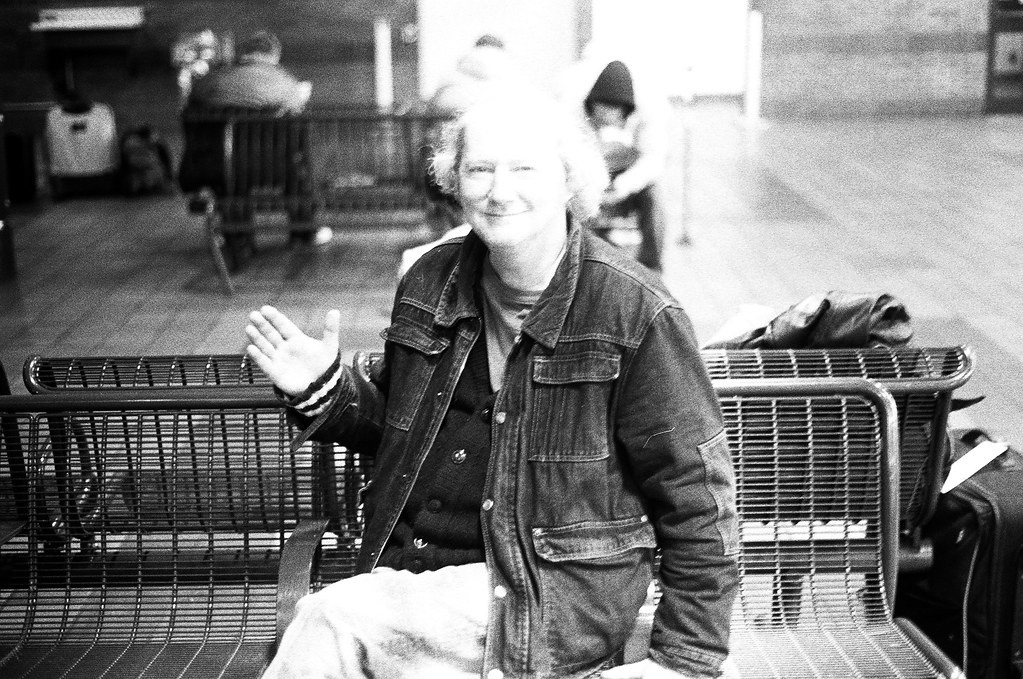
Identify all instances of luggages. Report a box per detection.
[894,428,1023,679]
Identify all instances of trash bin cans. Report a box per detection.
[983,1,1023,113]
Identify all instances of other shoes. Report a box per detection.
[293,226,332,247]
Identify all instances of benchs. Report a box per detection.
[202,104,466,294]
[1,346,977,679]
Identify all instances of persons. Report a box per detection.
[175,32,333,272]
[243,70,737,678]
[419,39,669,271]
